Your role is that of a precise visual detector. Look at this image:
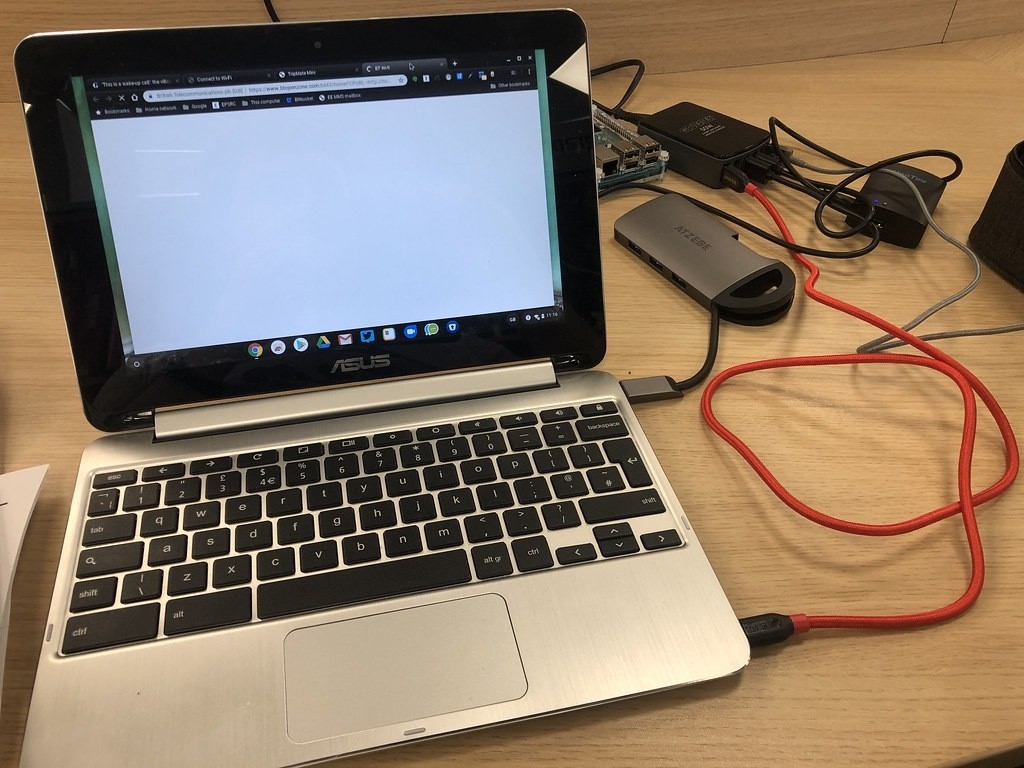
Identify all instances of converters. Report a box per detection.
[845,160,947,249]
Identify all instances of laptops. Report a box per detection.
[13,6,751,768]
